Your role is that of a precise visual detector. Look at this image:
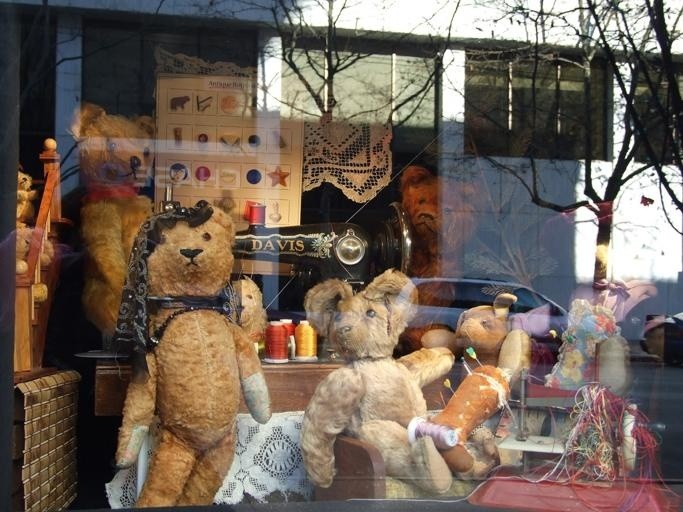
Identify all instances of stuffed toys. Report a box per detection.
[114,202,272,509]
[230,276,269,345]
[457,294,517,430]
[15,170,55,275]
[392,167,479,356]
[297,267,496,494]
[70,101,155,330]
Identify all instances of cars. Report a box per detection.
[638,309,683,363]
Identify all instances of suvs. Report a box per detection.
[382,273,616,366]
